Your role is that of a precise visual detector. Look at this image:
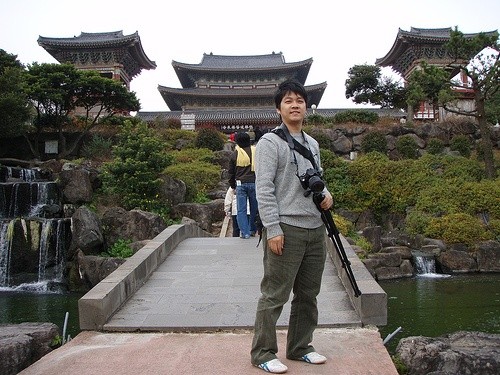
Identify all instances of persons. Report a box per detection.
[224,177,250,237]
[229,133,257,239]
[400,116,406,124]
[229,123,263,147]
[250,80,334,373]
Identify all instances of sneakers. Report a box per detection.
[254,359,289,373]
[297,351,327,364]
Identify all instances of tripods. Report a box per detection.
[313,192,361,297]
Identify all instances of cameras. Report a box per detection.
[300,168,324,193]
